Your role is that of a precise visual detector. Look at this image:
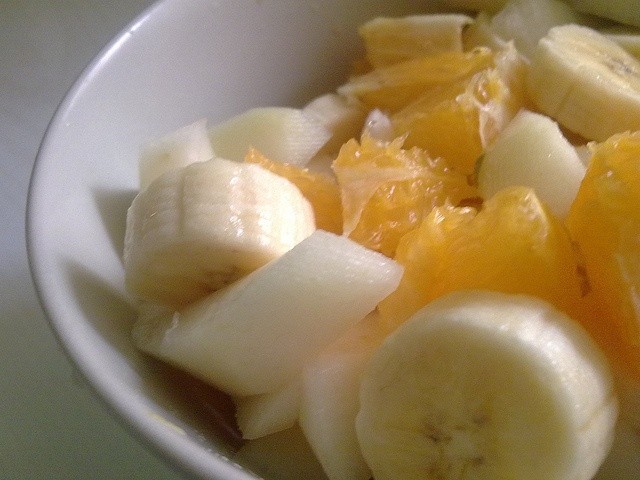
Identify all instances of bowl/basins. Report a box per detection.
[24,1,640,480]
